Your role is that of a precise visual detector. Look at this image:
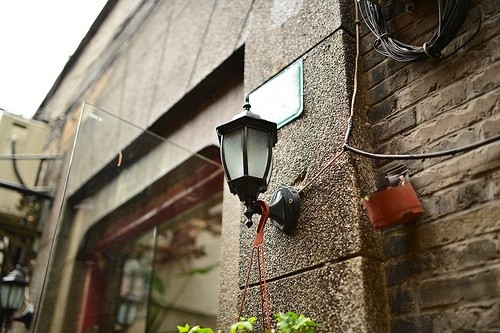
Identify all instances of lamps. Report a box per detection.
[213,89,302,242]
[0,264,35,331]
